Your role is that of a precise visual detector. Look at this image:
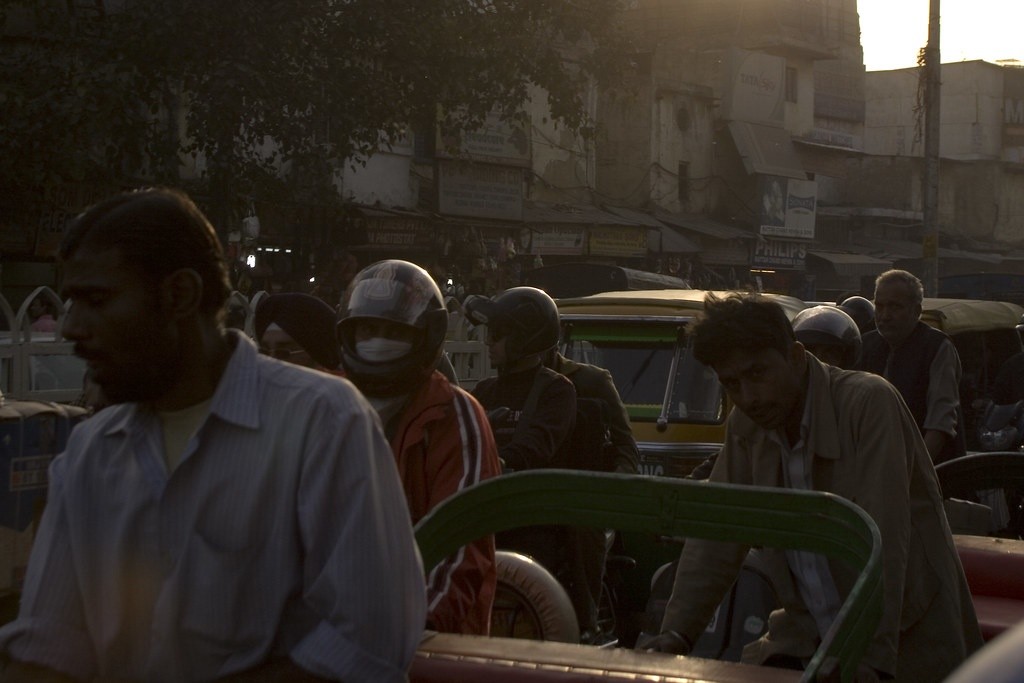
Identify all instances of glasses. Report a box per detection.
[488,327,508,342]
[258,348,305,360]
[801,340,847,366]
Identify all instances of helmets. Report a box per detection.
[840,296,873,326]
[488,287,560,360]
[336,259,449,399]
[792,306,862,343]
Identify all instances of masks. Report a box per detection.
[355,337,414,365]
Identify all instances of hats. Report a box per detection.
[255,294,340,370]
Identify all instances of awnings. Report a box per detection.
[646,225,701,254]
[654,213,768,243]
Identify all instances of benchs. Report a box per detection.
[933,451,1024,640]
[407,468,885,683]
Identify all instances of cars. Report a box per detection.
[857,293,1022,390]
[548,290,814,484]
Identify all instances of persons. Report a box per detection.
[639,270,982,682]
[1,191,646,683]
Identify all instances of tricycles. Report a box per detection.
[394,468,883,683]
[927,450,1024,647]
